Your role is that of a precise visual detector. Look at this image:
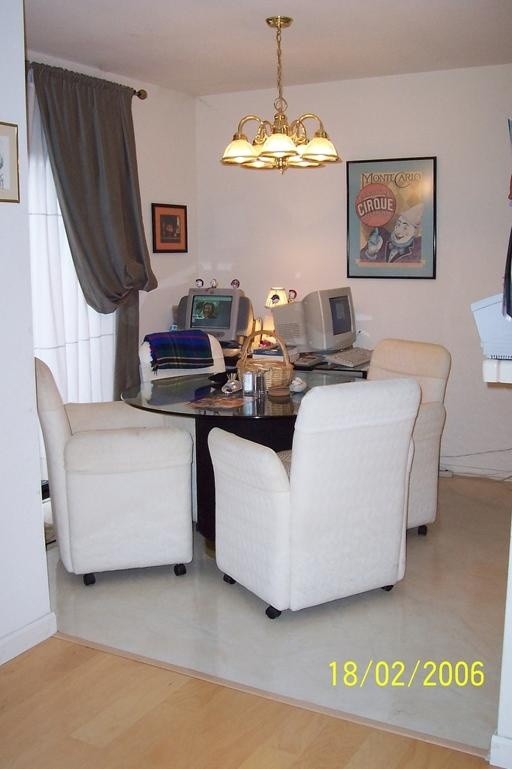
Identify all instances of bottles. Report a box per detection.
[243,368,264,395]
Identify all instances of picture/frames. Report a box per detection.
[0,120,21,204]
[345,155,438,280]
[151,202,189,254]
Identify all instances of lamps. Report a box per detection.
[264,286,288,309]
[219,15,342,174]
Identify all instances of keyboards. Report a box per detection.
[324,347,373,367]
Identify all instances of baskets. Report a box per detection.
[236,330,295,392]
[233,392,294,416]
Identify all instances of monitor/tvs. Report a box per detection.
[271,287,356,354]
[185,288,244,348]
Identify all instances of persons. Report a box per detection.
[201,301,220,320]
[358,201,423,265]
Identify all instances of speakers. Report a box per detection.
[177,296,188,331]
[237,296,254,336]
